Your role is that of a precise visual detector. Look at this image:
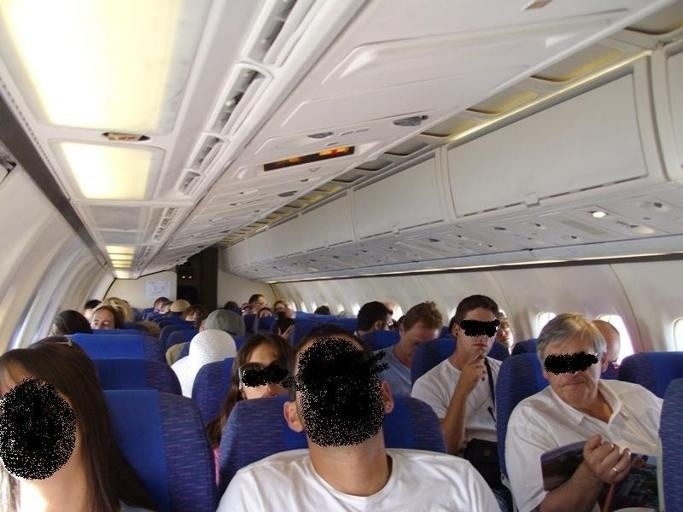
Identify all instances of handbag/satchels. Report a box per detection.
[538,441,656,511]
[464,438,500,470]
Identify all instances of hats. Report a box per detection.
[242,303,249,310]
[171,329,239,398]
[203,309,246,339]
[170,299,190,313]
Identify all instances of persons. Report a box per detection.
[2,292,662,511]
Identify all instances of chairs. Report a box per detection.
[60,308,682,511]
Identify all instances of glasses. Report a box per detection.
[255,303,267,306]
[385,320,394,330]
